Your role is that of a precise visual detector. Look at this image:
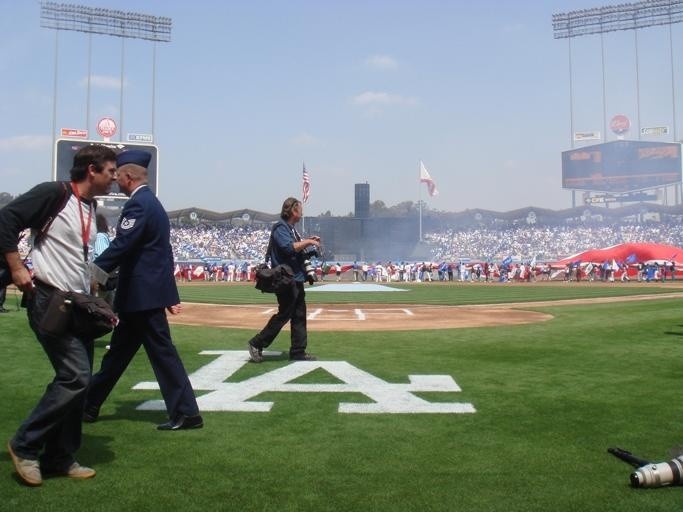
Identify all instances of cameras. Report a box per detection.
[298,250,318,285]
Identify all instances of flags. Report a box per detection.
[420,161,438,196]
[302,164,311,203]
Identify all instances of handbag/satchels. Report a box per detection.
[256,264,296,293]
[39,289,118,342]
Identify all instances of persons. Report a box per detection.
[95,214,117,304]
[17,223,274,281]
[1,144,120,485]
[82,151,203,431]
[0,254,11,313]
[312,223,682,282]
[248,197,321,362]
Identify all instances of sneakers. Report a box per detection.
[65,462,96,478]
[247,343,263,363]
[298,354,317,361]
[8,441,43,485]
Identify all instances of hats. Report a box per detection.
[115,150,151,167]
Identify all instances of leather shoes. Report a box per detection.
[83,408,100,423]
[157,413,203,431]
